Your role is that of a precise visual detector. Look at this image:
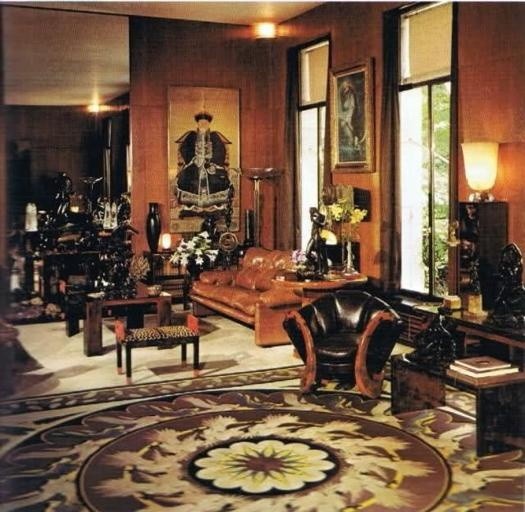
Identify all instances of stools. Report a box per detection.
[112,314,202,381]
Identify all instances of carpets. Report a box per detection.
[0,362,524,511]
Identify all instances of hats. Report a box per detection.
[194,111,212,122]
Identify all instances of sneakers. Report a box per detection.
[184,203,195,211]
[213,202,224,210]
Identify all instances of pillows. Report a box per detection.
[234,265,291,292]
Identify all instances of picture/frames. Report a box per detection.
[327,57,378,177]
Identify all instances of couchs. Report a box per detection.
[189,246,302,348]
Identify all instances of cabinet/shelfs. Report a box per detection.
[142,249,187,301]
[457,200,510,296]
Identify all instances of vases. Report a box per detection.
[146,202,163,253]
[182,262,199,310]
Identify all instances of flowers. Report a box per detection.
[319,197,368,239]
[168,231,218,269]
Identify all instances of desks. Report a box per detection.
[270,272,369,358]
[412,306,524,363]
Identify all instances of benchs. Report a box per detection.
[390,354,525,458]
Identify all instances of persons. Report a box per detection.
[174,111,233,210]
[338,76,356,143]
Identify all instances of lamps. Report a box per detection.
[460,141,500,202]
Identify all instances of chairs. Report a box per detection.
[283,289,405,401]
